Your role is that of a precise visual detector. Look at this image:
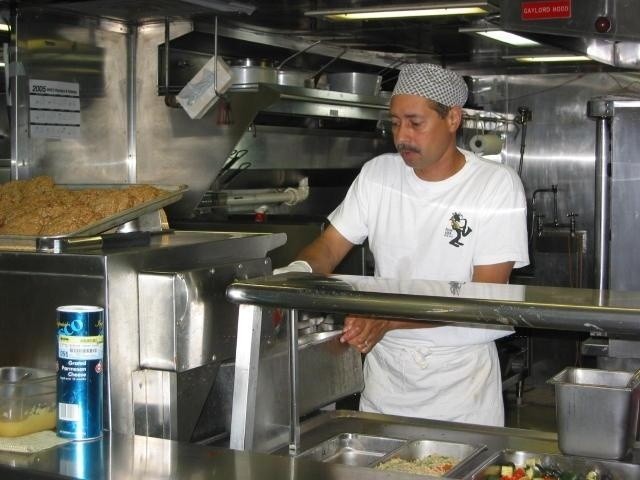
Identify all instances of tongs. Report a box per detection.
[33,230,151,256]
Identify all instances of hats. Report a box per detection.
[390,61,470,109]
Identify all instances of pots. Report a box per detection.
[327,57,402,96]
[276,49,347,88]
[227,38,321,88]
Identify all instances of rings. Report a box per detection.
[365,340,369,347]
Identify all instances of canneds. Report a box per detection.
[59,438,104,480]
[57,304,104,441]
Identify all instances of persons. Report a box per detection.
[271,62,530,427]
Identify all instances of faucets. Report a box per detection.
[532,184,557,217]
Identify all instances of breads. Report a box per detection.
[0,175,166,236]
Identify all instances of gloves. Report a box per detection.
[271,259,314,276]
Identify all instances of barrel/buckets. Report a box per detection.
[327,72,378,95]
